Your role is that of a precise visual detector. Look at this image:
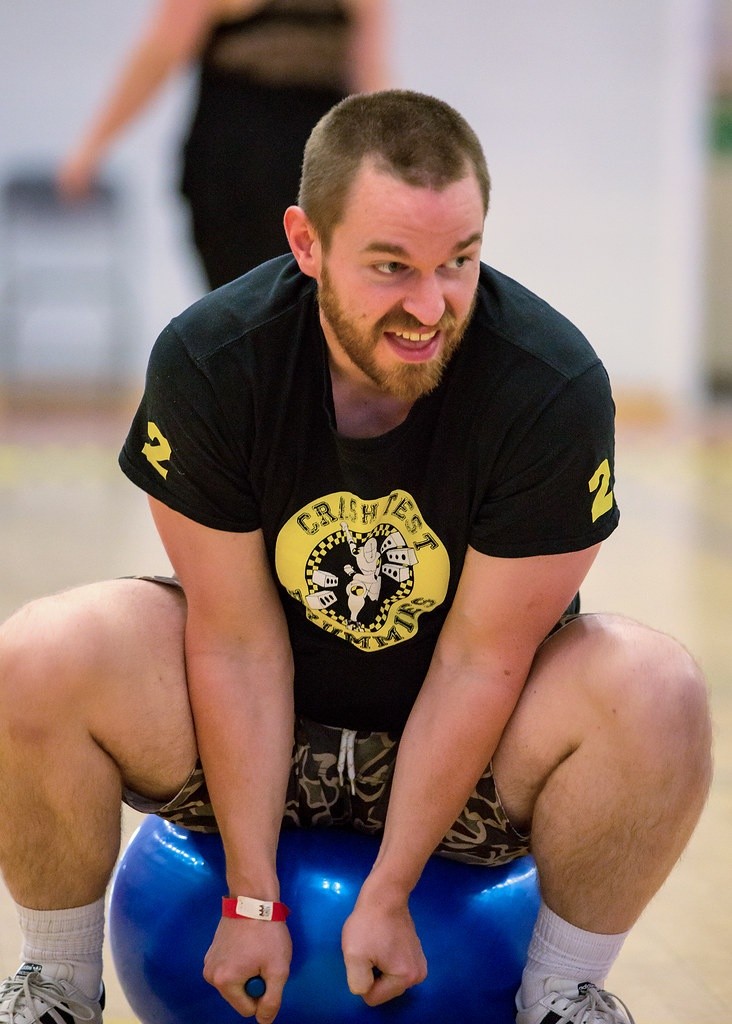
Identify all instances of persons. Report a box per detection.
[0,86,715,1021]
[63,0,393,294]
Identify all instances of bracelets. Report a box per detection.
[218,888,291,923]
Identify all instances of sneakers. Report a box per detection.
[515,983,635,1024]
[1,962,106,1024]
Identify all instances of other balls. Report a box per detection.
[112,814,543,1024]
